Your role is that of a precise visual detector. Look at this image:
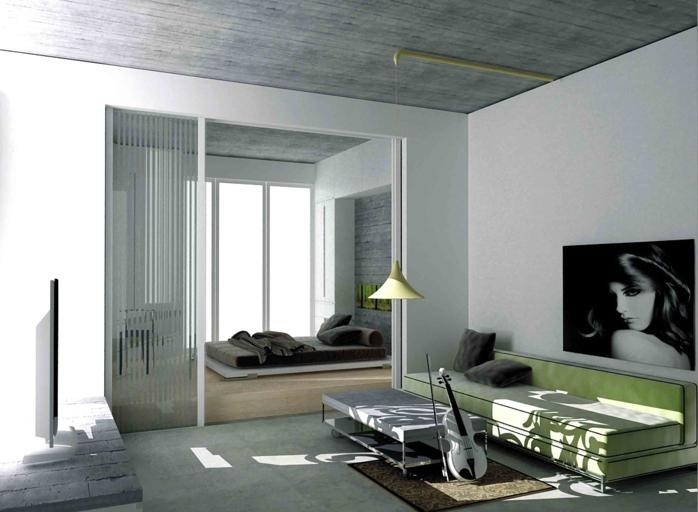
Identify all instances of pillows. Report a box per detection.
[359,328,384,347]
[317,314,352,337]
[453,329,496,372]
[318,326,361,345]
[464,359,532,388]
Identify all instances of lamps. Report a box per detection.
[367,46,558,299]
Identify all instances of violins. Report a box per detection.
[436,367,488,483]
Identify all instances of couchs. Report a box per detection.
[404,349,698,494]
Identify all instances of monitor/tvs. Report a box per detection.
[18,279,77,467]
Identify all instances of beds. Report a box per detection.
[205,337,392,379]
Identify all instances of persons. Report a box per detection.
[572,241,693,369]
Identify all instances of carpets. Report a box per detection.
[347,457,557,512]
